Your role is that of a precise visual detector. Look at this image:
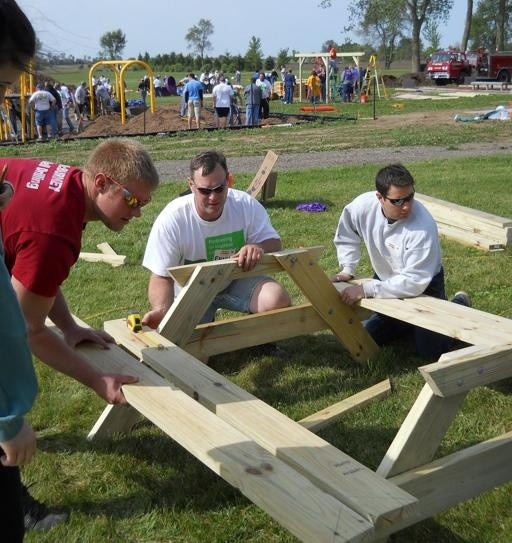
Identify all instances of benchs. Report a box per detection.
[333,276,512,347]
[469,80,509,91]
[44,309,419,543]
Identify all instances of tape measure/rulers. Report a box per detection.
[126,311,164,349]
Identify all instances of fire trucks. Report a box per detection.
[426,49,512,84]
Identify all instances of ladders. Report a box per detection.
[361,54,387,99]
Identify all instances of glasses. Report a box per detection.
[191,173,231,195]
[106,172,153,210]
[383,183,416,206]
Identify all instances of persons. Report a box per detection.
[138,69,273,130]
[18,474,70,535]
[1,132,160,408]
[272,60,369,104]
[1,0,41,539]
[141,149,293,360]
[6,76,113,144]
[322,160,471,358]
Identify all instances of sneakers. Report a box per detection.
[447,289,472,350]
[20,485,72,533]
[251,342,292,361]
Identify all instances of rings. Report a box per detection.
[336,275,340,281]
